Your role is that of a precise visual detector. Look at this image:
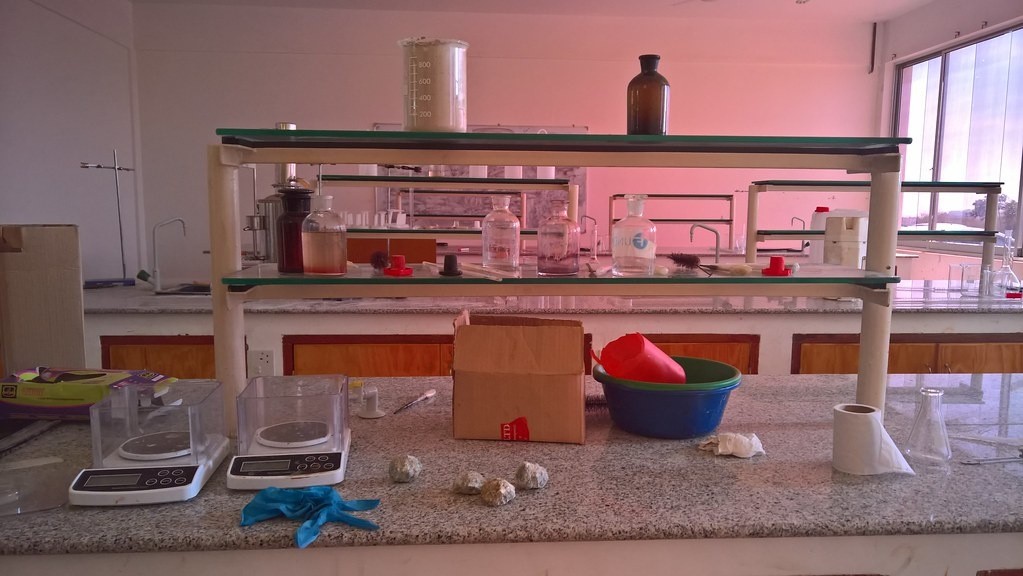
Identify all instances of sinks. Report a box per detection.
[154,283,211,295]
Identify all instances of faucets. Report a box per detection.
[580,215,597,224]
[689,224,720,262]
[136,217,185,292]
[791,216,804,230]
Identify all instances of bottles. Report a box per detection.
[989,231,1020,298]
[301,196,347,274]
[538,200,579,275]
[627,54,669,134]
[276,188,314,274]
[906,388,951,462]
[612,195,657,277]
[482,195,520,266]
[810,205,869,265]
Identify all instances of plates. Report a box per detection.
[385,267,413,275]
[438,270,462,275]
[761,267,791,276]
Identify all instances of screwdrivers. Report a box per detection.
[393,389,436,414]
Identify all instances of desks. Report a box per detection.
[207,127,912,434]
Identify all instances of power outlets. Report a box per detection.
[247,350,274,378]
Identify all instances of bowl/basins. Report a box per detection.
[594,355,742,438]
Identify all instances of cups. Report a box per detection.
[538,166,555,179]
[958,264,989,297]
[469,166,487,178]
[770,256,785,271]
[475,221,480,227]
[503,166,522,179]
[444,254,457,273]
[398,36,469,132]
[390,255,405,271]
[429,165,444,178]
[590,333,686,384]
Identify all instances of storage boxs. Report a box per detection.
[450,308,586,446]
[0,365,183,424]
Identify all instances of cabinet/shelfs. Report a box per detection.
[281,335,454,378]
[638,333,760,377]
[792,333,1022,375]
[100,335,216,381]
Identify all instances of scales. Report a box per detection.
[226,419,353,490]
[68,428,236,506]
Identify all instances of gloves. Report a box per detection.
[240,486,381,549]
